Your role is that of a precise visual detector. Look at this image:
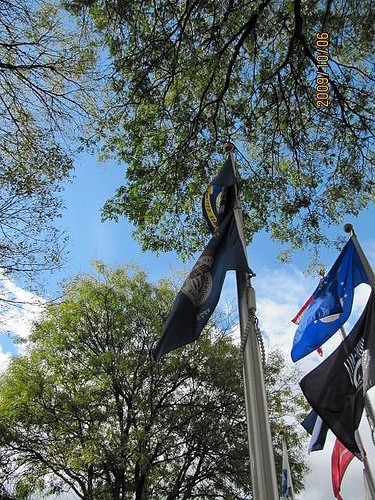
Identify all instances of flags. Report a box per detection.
[289,232,375,364]
[149,149,258,361]
[299,409,331,455]
[298,287,375,463]
[279,437,296,500]
[330,439,356,500]
